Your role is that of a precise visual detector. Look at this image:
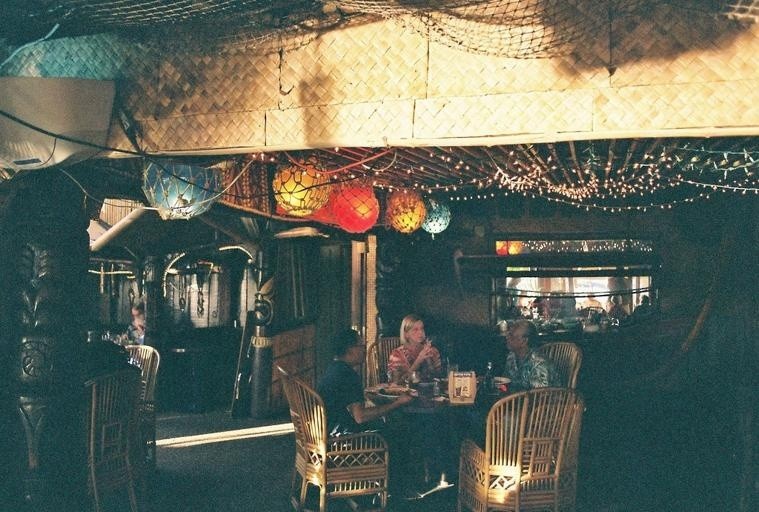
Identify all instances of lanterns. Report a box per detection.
[270,156,329,218]
[422,195,452,235]
[142,158,218,219]
[385,186,426,236]
[331,176,380,236]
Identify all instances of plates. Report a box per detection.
[376,388,400,398]
[494,376,511,385]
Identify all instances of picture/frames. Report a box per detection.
[448,370,478,405]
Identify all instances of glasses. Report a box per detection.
[353,344,367,349]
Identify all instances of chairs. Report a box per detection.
[125,345,161,467]
[85,365,142,512]
[276,364,391,511]
[364,335,401,407]
[537,340,584,390]
[457,387,585,512]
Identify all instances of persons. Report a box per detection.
[497,319,567,399]
[386,312,446,388]
[586,291,649,324]
[314,326,412,506]
[118,300,146,347]
